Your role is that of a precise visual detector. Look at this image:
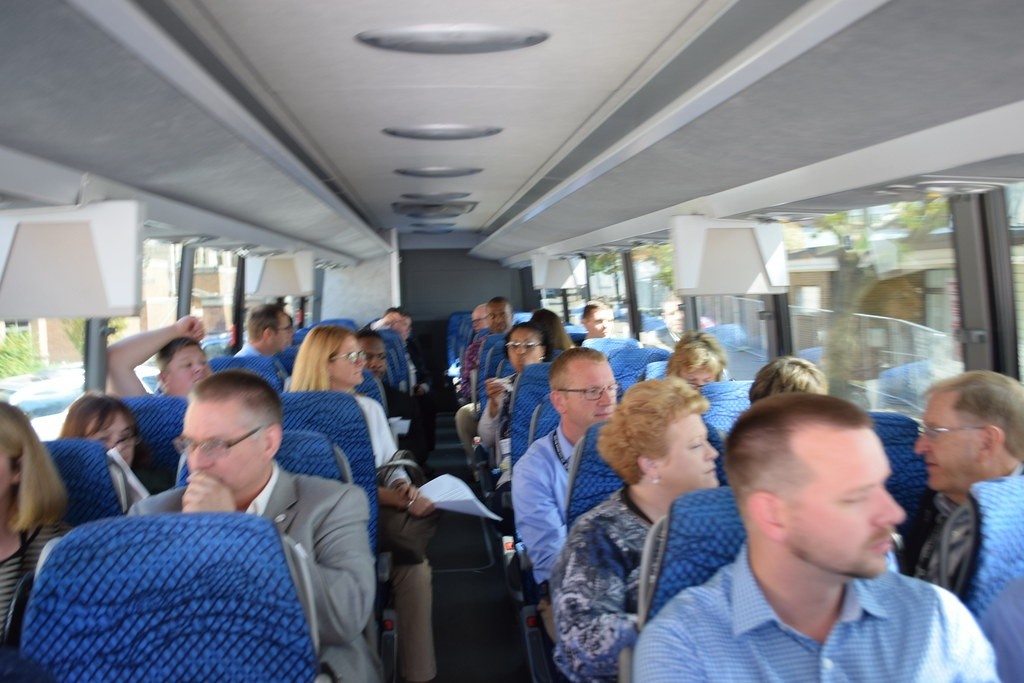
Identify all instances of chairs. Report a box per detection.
[322,320,357,332]
[174,429,353,488]
[120,396,193,493]
[41,439,128,526]
[381,332,408,381]
[210,356,287,393]
[446,311,1024,683]
[274,345,300,377]
[2,511,321,683]
[385,347,401,383]
[292,328,311,345]
[278,390,398,683]
[284,369,387,421]
[382,365,395,390]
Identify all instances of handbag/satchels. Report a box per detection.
[376,449,437,564]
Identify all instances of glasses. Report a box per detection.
[171,424,263,461]
[915,422,988,442]
[506,341,543,353]
[97,424,140,453]
[326,349,366,364]
[558,383,619,401]
[274,321,293,335]
[469,317,485,326]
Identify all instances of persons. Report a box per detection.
[106,316,215,397]
[234,305,440,683]
[642,295,686,352]
[748,356,831,404]
[126,367,376,683]
[461,303,489,402]
[886,370,1024,608]
[513,346,617,640]
[478,322,546,535]
[0,401,72,683]
[454,297,513,470]
[574,300,615,348]
[56,391,173,511]
[551,376,720,683]
[633,392,1000,683]
[665,329,729,392]
[530,309,575,362]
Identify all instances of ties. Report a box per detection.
[273,361,285,391]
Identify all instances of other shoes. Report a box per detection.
[507,553,522,591]
